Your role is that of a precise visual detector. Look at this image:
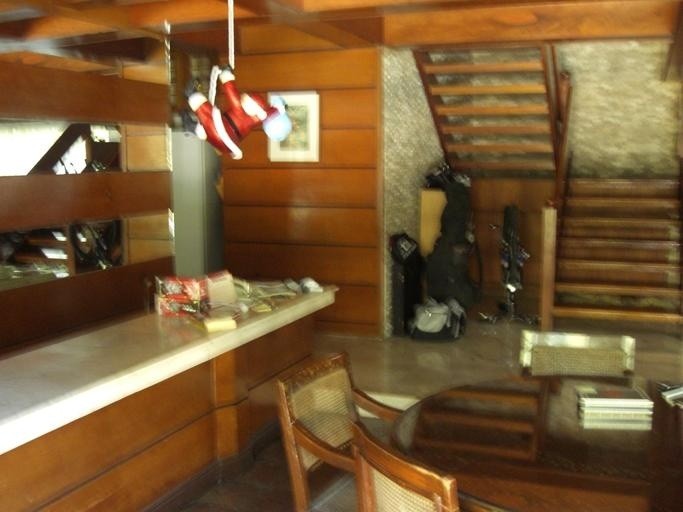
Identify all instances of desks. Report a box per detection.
[391,375,683,511]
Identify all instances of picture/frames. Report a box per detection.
[267,91,321,163]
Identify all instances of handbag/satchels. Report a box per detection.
[407,297,467,340]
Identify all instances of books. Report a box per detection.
[558,375,683,431]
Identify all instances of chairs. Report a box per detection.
[276,351,405,511]
[354,420,459,511]
[520,329,636,380]
